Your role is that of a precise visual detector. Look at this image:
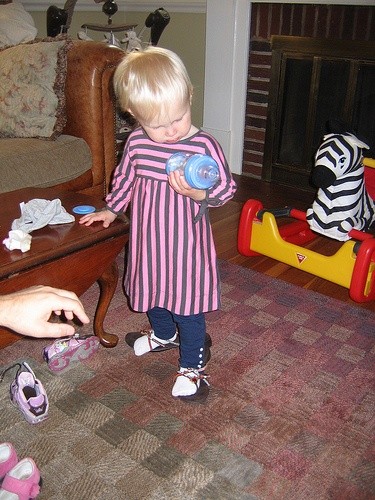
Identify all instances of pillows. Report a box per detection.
[0,32,73,140]
[0,1,38,47]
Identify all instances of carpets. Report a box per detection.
[0,250,375,500]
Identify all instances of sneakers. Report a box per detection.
[9,362,49,425]
[42,334,100,375]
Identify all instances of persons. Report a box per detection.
[0,284,90,337]
[79,46,238,398]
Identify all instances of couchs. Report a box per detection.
[0,40,133,198]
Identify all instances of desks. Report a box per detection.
[80,23,138,35]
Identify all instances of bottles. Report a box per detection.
[165,152,220,190]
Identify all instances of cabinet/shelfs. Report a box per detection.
[261,35,375,190]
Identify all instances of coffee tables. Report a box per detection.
[0,187,130,349]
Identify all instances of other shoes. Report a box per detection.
[0,442,18,478]
[0,457,40,500]
